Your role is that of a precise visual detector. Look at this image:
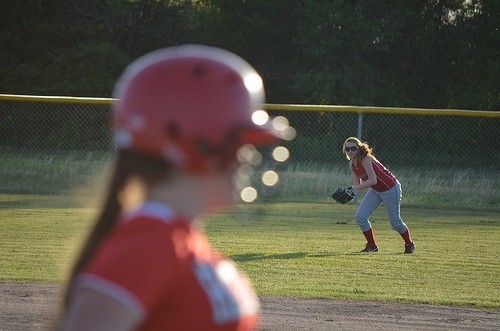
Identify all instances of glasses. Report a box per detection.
[345,146,359,152]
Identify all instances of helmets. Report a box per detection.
[112,45,297,174]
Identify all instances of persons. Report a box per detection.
[332,137,415,253]
[64,44,295,331]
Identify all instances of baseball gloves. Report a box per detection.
[332,186,357,204]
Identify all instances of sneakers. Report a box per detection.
[404,242,415,254]
[360,246,378,252]
